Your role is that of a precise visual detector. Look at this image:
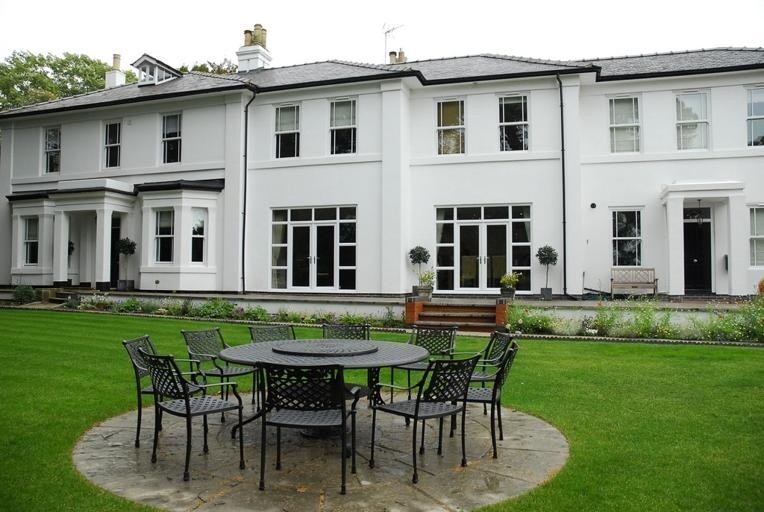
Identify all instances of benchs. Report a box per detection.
[608,265,660,302]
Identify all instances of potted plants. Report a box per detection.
[537,246,559,300]
[416,269,436,300]
[500,272,521,297]
[113,238,138,292]
[408,246,430,289]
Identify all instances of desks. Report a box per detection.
[220,337,430,437]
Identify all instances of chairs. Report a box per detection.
[256,360,357,496]
[437,343,520,460]
[322,324,371,399]
[121,334,208,448]
[248,325,298,405]
[452,332,513,429]
[181,326,257,422]
[137,347,245,481]
[370,354,484,484]
[388,325,458,403]
